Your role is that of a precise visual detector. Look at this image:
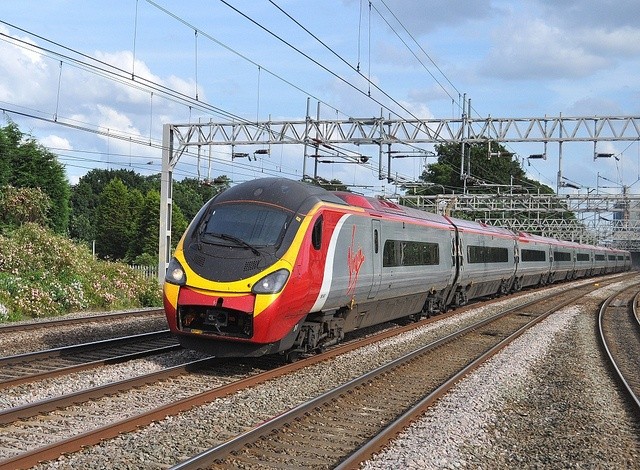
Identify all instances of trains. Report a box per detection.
[163,177,631,363]
